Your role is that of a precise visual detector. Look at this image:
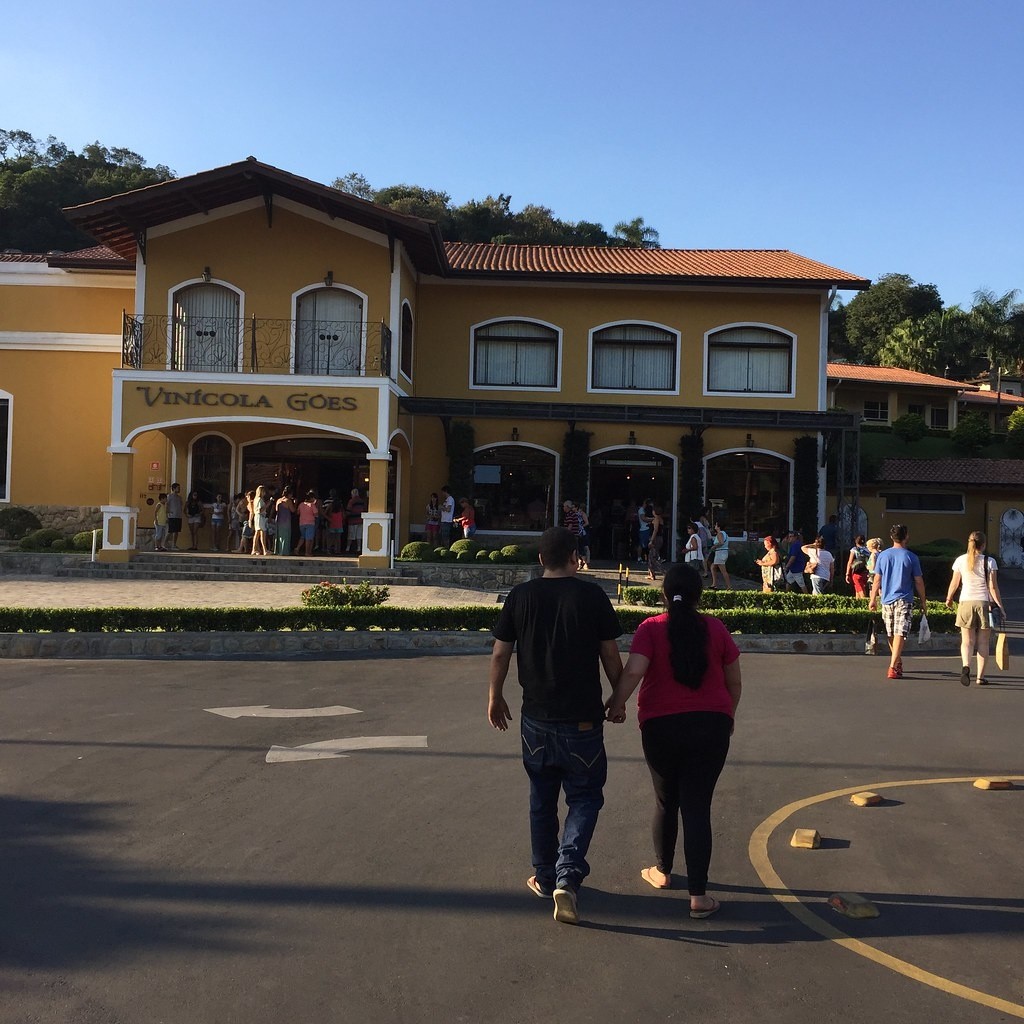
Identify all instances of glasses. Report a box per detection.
[194,494,197,496]
[687,527,692,529]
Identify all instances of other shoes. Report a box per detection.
[153,544,360,556]
[888,656,902,677]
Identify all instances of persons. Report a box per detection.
[869,525,928,678]
[485,526,626,925]
[425,484,476,549]
[946,530,1007,687]
[637,498,666,579]
[154,482,368,556]
[819,513,840,558]
[564,501,590,570]
[683,510,732,591]
[753,530,883,600]
[606,565,743,917]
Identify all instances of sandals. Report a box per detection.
[708,586,718,590]
[959,665,971,687]
[975,677,990,688]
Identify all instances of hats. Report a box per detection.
[351,489,359,497]
[789,529,800,540]
[564,501,573,508]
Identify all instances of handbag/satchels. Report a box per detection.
[851,556,867,572]
[804,548,818,574]
[995,618,1010,671]
[580,527,590,546]
[708,550,715,564]
[987,606,1004,629]
[689,559,703,570]
[244,521,254,538]
[771,582,792,592]
[917,614,932,644]
[864,607,880,655]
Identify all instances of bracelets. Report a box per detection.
[947,595,952,601]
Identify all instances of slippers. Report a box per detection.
[553,887,577,922]
[640,867,671,889]
[527,875,554,899]
[691,893,721,919]
